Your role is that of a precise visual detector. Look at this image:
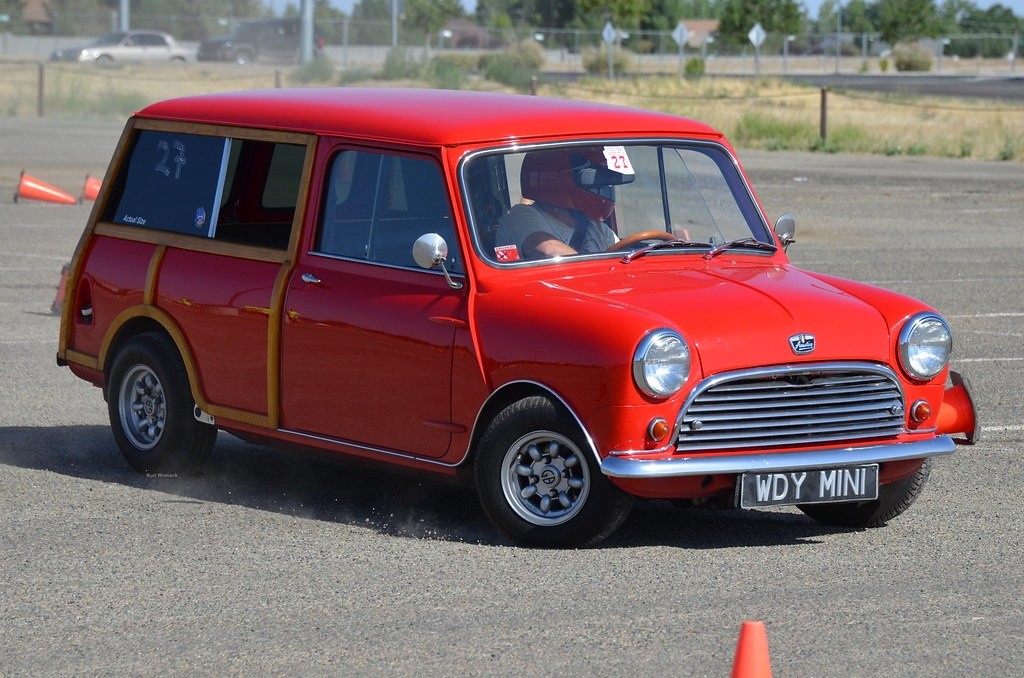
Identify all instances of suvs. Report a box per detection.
[197,18,327,66]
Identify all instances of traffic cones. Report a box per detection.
[15,170,76,205]
[51,266,70,317]
[730,621,774,678]
[80,174,102,204]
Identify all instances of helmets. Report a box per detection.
[520,145,635,222]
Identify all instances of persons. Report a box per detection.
[493,142,636,263]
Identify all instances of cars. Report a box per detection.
[55,86,981,552]
[51,29,193,70]
